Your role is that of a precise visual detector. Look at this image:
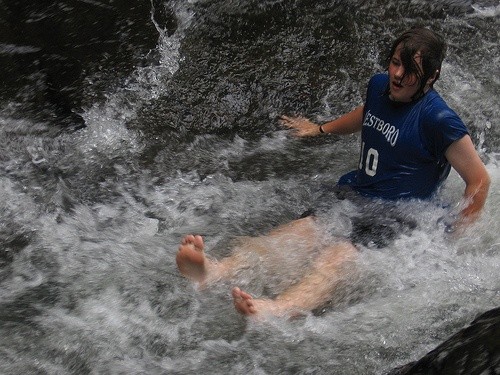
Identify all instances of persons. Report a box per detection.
[175,26,490,319]
